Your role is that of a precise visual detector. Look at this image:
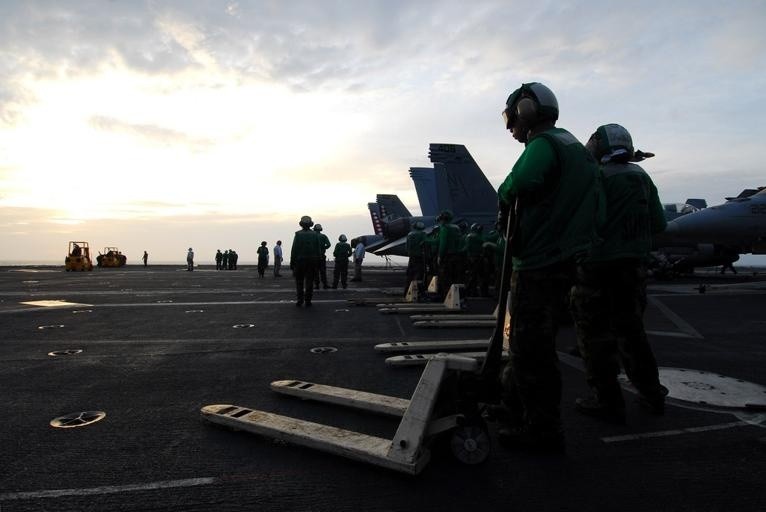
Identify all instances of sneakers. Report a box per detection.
[259,273,282,278]
[575,393,627,424]
[313,284,348,290]
[490,410,564,452]
[635,387,670,415]
[294,300,313,306]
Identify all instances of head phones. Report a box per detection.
[471,223,481,232]
[517,84,538,122]
[601,148,628,163]
[300,221,313,226]
[443,210,449,221]
[413,225,425,230]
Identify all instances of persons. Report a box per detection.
[289,217,320,308]
[274,241,283,277]
[257,242,269,278]
[498,83,606,464]
[216,249,237,270]
[186,248,193,271]
[144,252,148,267]
[576,123,666,414]
[350,237,367,282]
[314,225,333,290]
[405,212,498,297]
[331,236,352,289]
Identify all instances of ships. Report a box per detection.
[0,258,766,512]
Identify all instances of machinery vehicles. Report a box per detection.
[95,244,131,267]
[64,239,94,270]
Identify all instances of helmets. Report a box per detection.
[313,224,323,232]
[299,216,314,228]
[338,235,347,242]
[591,124,635,162]
[415,209,484,234]
[504,81,560,138]
